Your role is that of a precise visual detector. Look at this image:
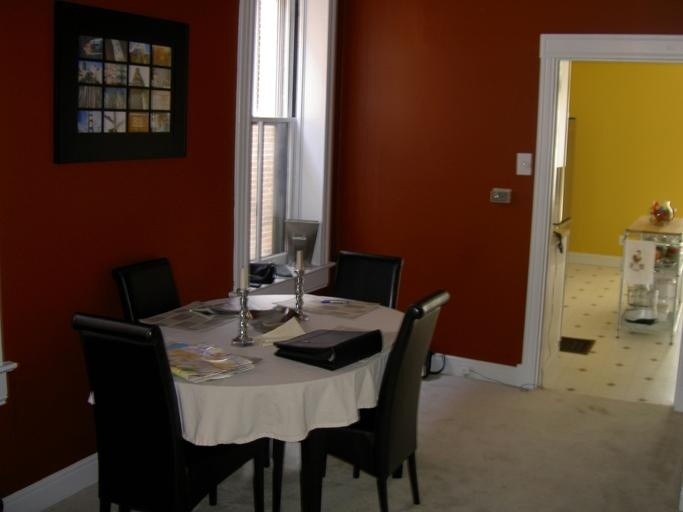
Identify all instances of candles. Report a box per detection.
[297,250,301,272]
[240,265,245,289]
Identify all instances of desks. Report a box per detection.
[616,215,682,345]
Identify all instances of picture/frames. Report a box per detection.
[52,0,188,164]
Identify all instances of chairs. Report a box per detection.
[331,251,402,308]
[272,290,451,511]
[73,314,264,512]
[112,257,180,324]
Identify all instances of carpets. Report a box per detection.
[45,374,683,512]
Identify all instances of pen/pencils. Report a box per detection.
[321,300,352,304]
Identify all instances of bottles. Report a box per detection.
[649,200,675,226]
